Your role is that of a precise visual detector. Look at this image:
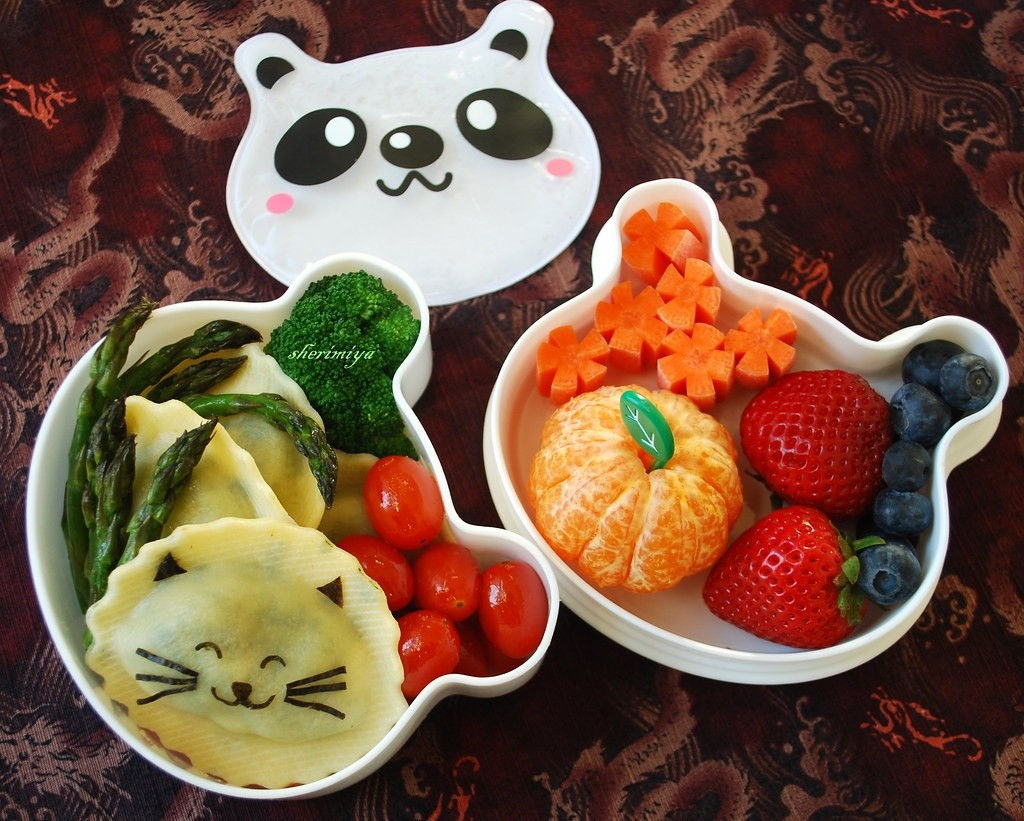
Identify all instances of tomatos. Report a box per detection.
[338,458,547,696]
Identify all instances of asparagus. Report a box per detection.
[60,283,339,648]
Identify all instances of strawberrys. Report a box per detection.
[738,368,894,517]
[700,504,886,649]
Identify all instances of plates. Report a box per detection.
[22,248,561,802]
[478,177,1012,689]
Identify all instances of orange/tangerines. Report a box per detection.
[529,384,742,591]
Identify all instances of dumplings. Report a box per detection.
[83,340,414,789]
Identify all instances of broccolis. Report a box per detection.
[267,268,416,461]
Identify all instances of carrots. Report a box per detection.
[536,202,797,410]
